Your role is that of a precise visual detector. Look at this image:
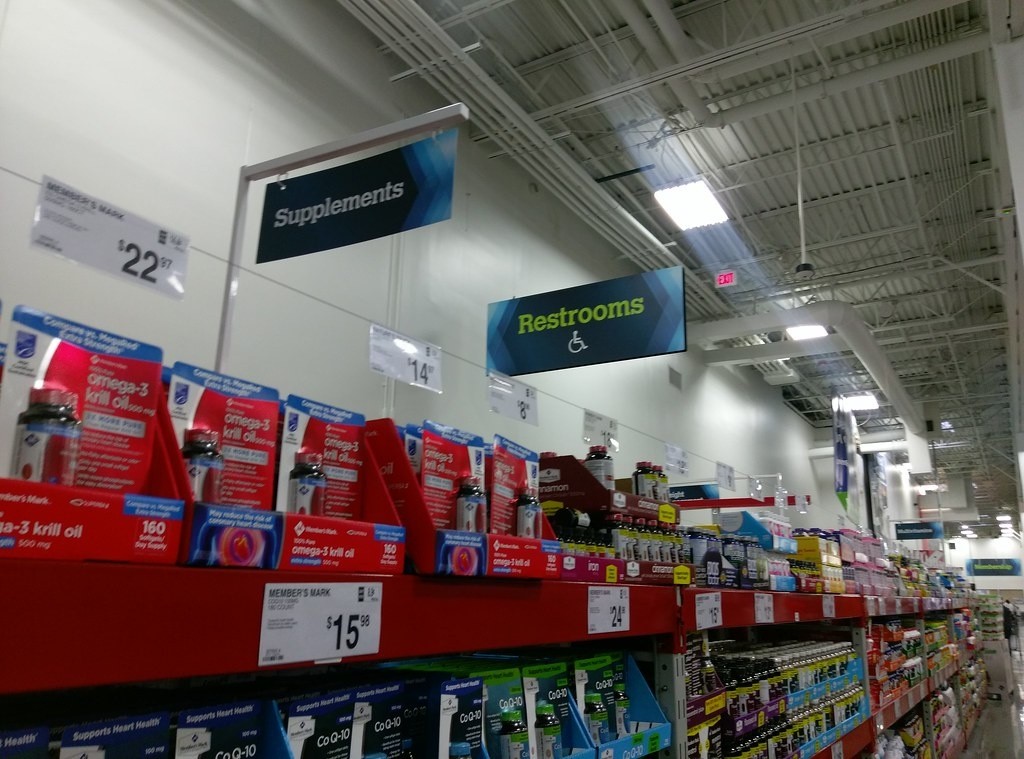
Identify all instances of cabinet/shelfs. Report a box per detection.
[685,584,988,759]
[0,557,684,759]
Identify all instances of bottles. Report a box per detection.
[584,693,609,745]
[710,641,866,759]
[401,739,414,759]
[180,429,224,504]
[456,476,486,533]
[585,445,616,490]
[556,513,762,569]
[614,684,630,739]
[632,462,658,500]
[786,559,820,579]
[516,487,542,539]
[9,389,81,486]
[652,465,667,501]
[449,742,472,759]
[288,451,326,516]
[499,710,530,759]
[534,704,562,759]
